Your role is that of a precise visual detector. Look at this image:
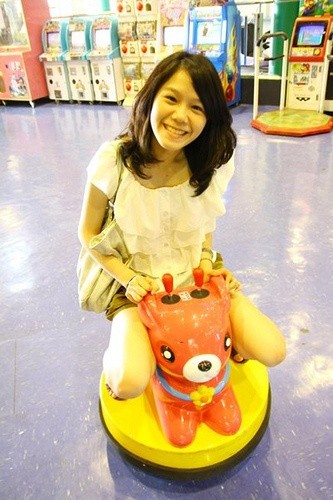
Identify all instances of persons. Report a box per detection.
[78,51,289,404]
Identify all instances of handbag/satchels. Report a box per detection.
[74,202,131,314]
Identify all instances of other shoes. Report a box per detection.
[229,347,247,363]
